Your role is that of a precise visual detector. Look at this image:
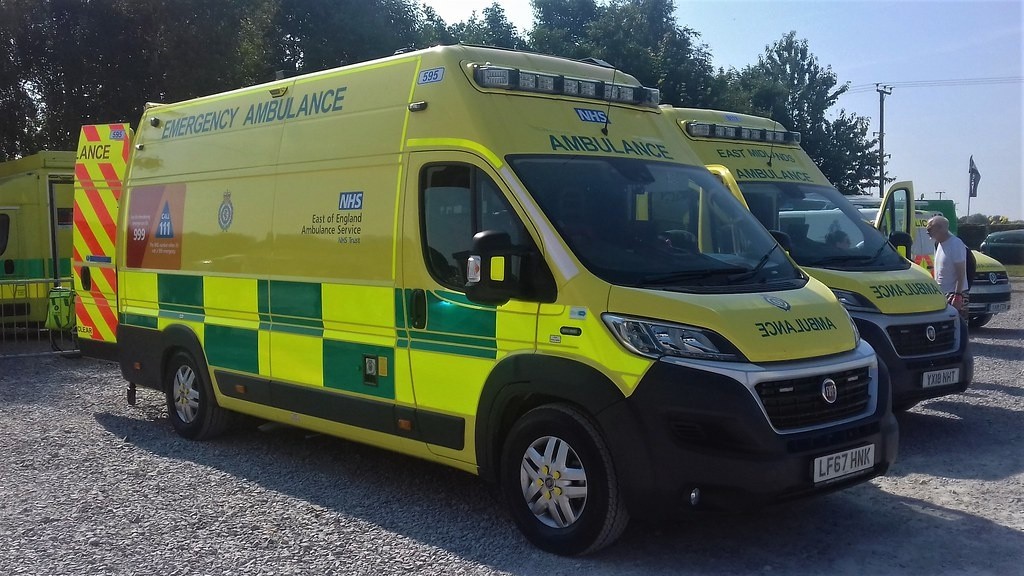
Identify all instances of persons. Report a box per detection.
[926,216,976,325]
[826,220,876,252]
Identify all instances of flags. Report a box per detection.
[968,157,981,198]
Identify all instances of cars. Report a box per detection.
[979,229,1024,265]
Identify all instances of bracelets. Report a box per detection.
[954,292,962,295]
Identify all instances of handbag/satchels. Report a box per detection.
[44,291,75,332]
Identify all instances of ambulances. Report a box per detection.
[69,45,896,559]
[777,208,1011,328]
[453,105,975,413]
[0,151,77,327]
